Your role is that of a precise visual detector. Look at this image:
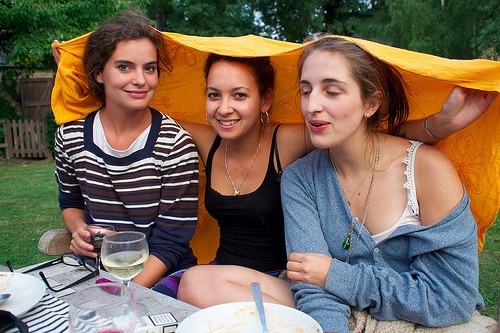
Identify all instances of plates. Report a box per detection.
[0,272,47,318]
[175,301,323,333]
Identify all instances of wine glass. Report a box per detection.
[100,230,149,319]
[84,224,115,280]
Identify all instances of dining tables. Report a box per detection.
[0,257,204,333]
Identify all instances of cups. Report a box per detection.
[68,283,137,333]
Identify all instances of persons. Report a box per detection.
[279,34,500,333]
[49,33,500,310]
[52,9,202,300]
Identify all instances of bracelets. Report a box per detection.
[423,112,447,143]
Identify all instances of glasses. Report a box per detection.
[6,251,101,293]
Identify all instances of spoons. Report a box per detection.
[0,294,11,300]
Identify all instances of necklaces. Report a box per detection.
[223,124,267,197]
[328,128,382,264]
